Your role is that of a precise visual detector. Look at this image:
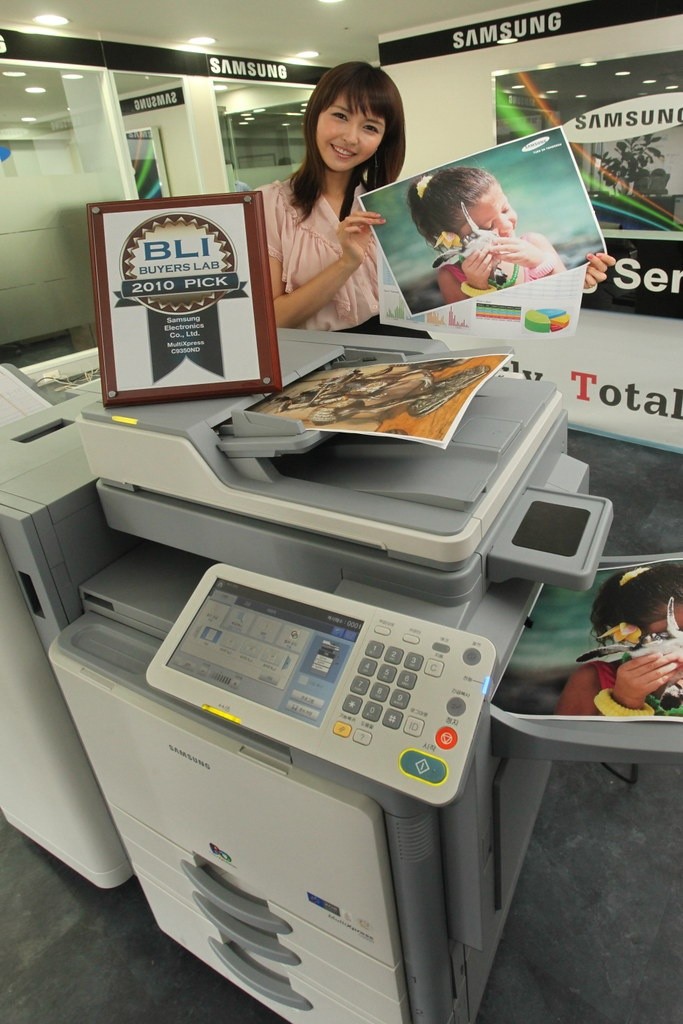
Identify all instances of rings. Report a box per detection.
[588,285,596,290]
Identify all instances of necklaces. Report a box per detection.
[459,253,519,290]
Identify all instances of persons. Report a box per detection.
[411,167,568,305]
[555,563,683,716]
[252,62,617,340]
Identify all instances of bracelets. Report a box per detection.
[461,281,497,297]
[594,689,655,717]
[528,252,558,279]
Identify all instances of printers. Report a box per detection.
[0,323,683,1024]
